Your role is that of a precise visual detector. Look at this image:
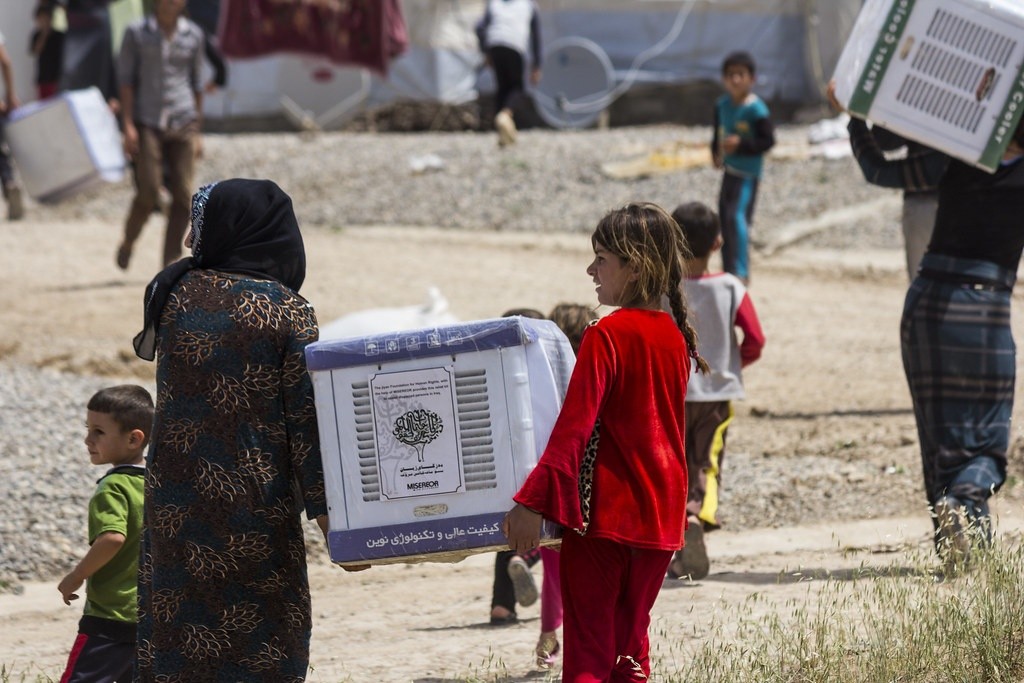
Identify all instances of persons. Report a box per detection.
[473,0,542,150]
[488,307,545,621]
[117,1,228,269]
[54,385,156,683]
[502,201,713,683]
[533,304,600,666]
[709,50,778,286]
[826,80,1023,576]
[658,199,766,582]
[60,0,121,114]
[0,30,25,221]
[27,0,65,99]
[134,180,371,683]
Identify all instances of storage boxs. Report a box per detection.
[305,315,578,566]
[2,85,132,205]
[831,0,1024,174]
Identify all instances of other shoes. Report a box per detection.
[496,113,517,147]
[491,613,517,624]
[8,185,23,220]
[118,245,130,268]
[936,496,971,564]
[535,640,560,672]
[508,555,538,606]
[678,516,709,580]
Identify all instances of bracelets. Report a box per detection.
[523,504,543,515]
